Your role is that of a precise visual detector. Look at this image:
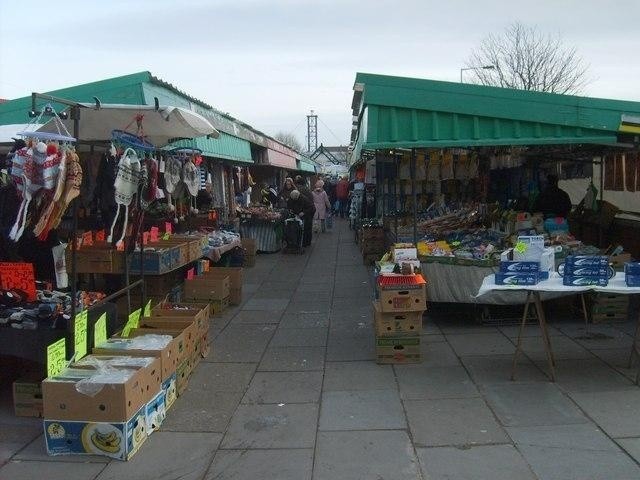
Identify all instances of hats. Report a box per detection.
[6,137,83,204]
[93,142,158,211]
[155,155,199,198]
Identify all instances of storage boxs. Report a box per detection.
[356,228,386,267]
[93,340,176,410]
[186,276,229,315]
[208,265,244,305]
[593,312,627,324]
[596,301,629,313]
[111,329,190,397]
[12,382,42,417]
[129,234,202,273]
[373,301,422,339]
[71,247,126,274]
[379,280,426,313]
[74,355,167,436]
[41,368,145,460]
[122,321,203,375]
[598,294,630,301]
[144,308,206,358]
[243,255,256,267]
[242,239,258,256]
[150,302,211,344]
[374,339,424,364]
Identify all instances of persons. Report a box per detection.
[530,173,571,219]
[281,172,349,247]
